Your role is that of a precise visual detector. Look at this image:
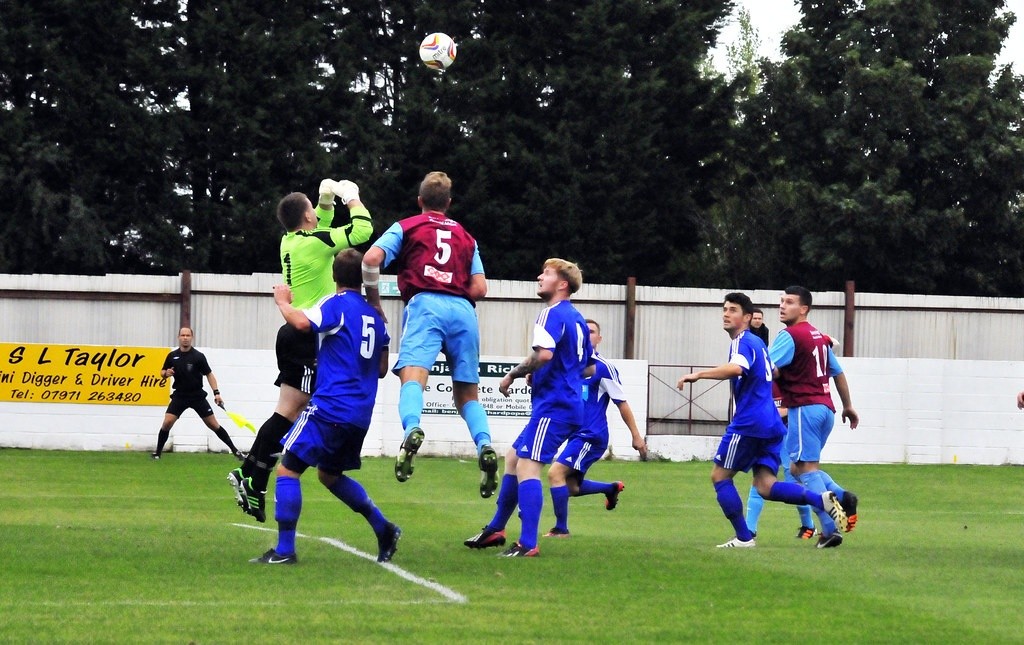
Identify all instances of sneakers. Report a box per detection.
[714,530,757,547]
[377,522,401,563]
[394,426,425,482]
[235,451,246,461]
[496,541,539,557]
[464,525,505,550]
[248,548,297,565]
[814,530,843,549]
[796,526,817,540]
[478,445,499,498]
[149,453,161,459]
[605,481,624,511]
[821,490,848,533]
[839,492,858,532]
[227,467,268,523]
[543,528,569,538]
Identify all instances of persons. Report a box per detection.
[768,286,859,547]
[226,178,374,527]
[540,320,646,539]
[464,257,595,557]
[747,309,768,347]
[248,248,401,567]
[151,326,247,460]
[677,293,848,549]
[362,171,500,498]
[745,375,816,541]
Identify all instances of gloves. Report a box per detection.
[318,179,359,205]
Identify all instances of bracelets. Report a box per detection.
[214,390,220,396]
[164,372,168,378]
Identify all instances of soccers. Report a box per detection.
[419,32,456,70]
